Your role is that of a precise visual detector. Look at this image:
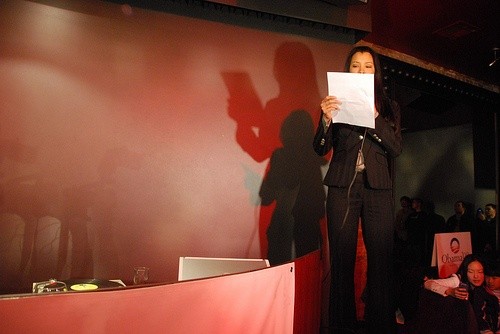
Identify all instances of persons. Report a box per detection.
[314,45,403,334]
[395,195,500,334]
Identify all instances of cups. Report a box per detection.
[35,281,69,293]
[134,267,150,283]
